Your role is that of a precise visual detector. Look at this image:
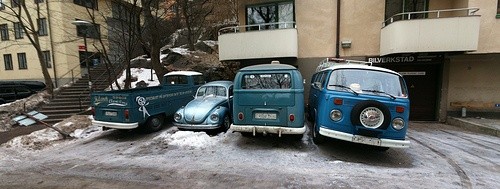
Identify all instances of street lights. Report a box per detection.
[71,20,94,93]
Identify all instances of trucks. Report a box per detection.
[89,70,206,134]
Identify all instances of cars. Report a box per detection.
[230,61,307,142]
[172,81,233,136]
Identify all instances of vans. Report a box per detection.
[307,57,411,152]
